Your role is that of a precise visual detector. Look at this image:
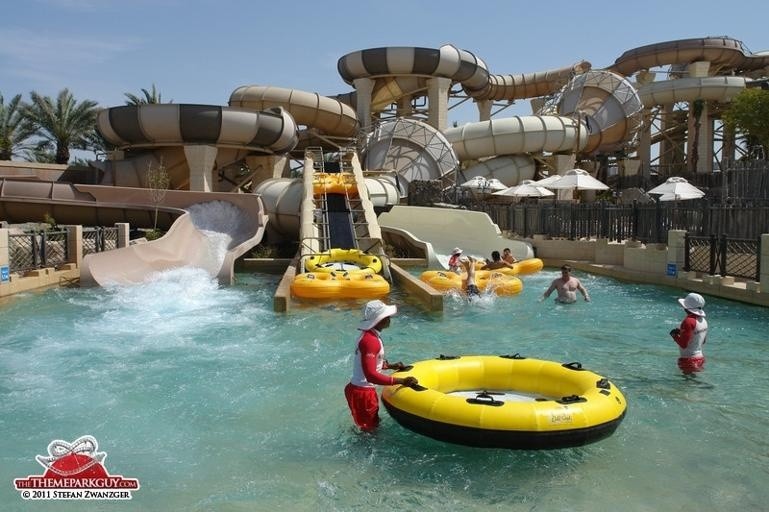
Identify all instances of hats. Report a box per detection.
[451,248,463,256]
[357,299,397,332]
[677,292,706,317]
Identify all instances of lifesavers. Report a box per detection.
[382,353,627,451]
[305,247,381,274]
[421,258,543,296]
[312,173,358,195]
[292,274,390,298]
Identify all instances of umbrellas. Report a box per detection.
[647,176,705,201]
[461,167,610,199]
[659,193,704,202]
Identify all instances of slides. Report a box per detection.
[0,35,769,290]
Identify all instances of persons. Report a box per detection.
[461,255,479,300]
[502,248,517,263]
[343,298,418,435]
[669,292,708,385]
[448,247,464,274]
[537,264,590,303]
[481,250,513,270]
